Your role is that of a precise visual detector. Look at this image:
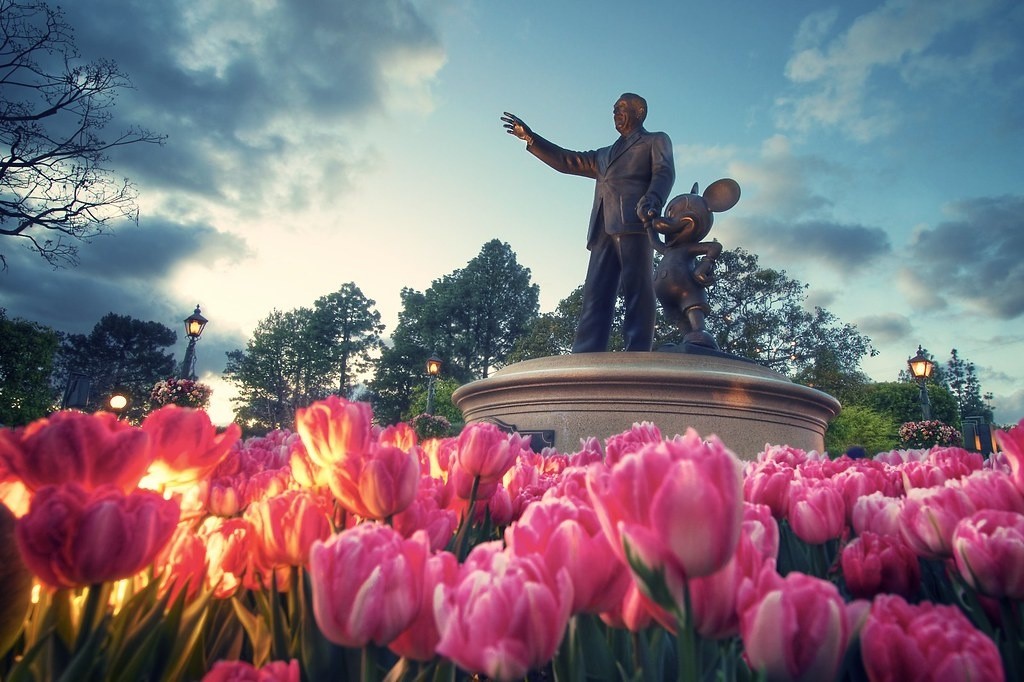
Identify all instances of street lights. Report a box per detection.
[179,304,208,380]
[425,349,442,418]
[907,342,933,422]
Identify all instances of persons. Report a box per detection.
[500,92,676,354]
